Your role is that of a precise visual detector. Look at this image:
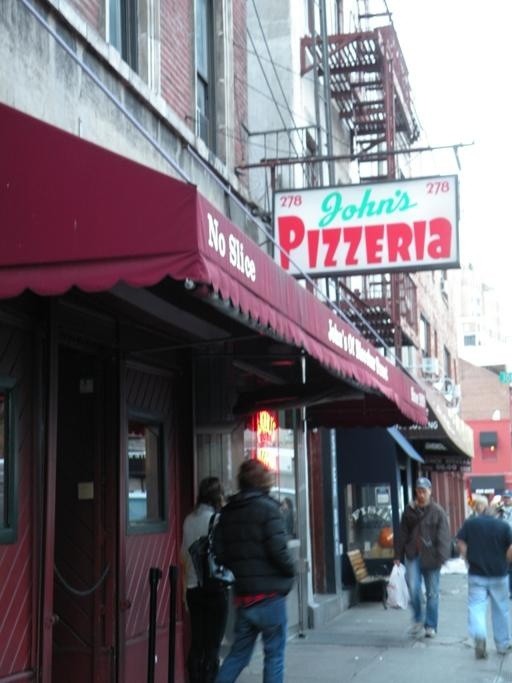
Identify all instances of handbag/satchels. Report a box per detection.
[189,511,236,593]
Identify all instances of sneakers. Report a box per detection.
[410,623,437,639]
[475,638,510,660]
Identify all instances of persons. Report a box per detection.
[212,459,296,682]
[475,496,487,517]
[456,495,512,660]
[175,476,236,682]
[395,478,450,637]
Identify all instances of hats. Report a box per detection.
[238,461,274,490]
[416,478,432,489]
[503,489,512,497]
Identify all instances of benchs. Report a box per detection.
[346,548,390,608]
